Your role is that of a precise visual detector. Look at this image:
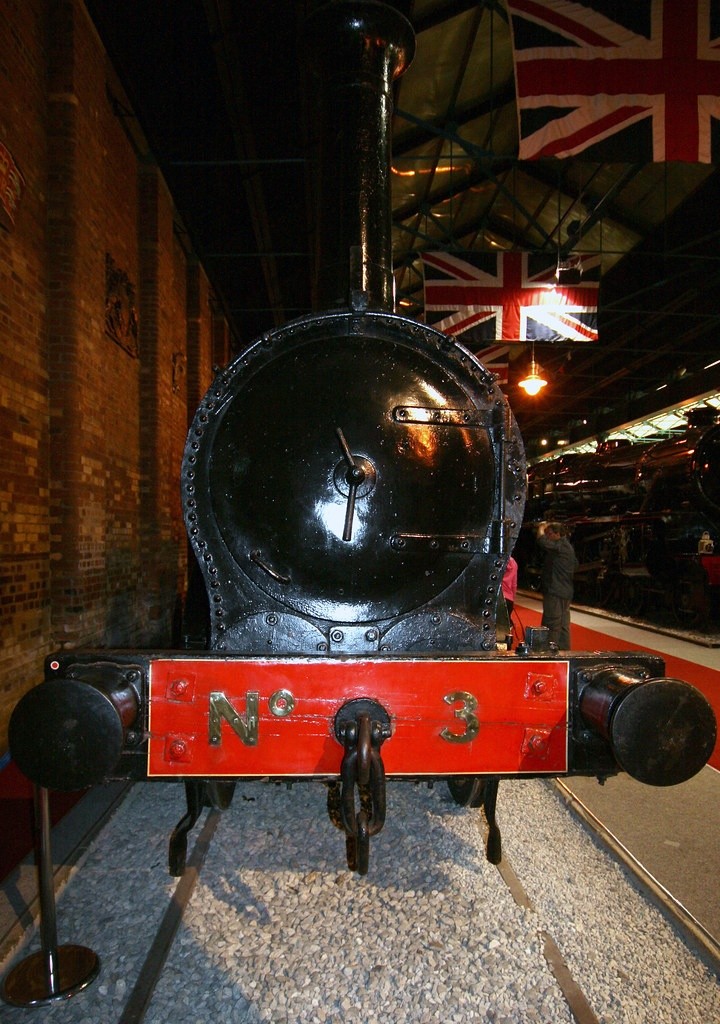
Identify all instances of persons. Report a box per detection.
[501,556,518,624]
[535,520,577,650]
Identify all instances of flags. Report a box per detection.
[418,247,603,345]
[508,0,720,164]
